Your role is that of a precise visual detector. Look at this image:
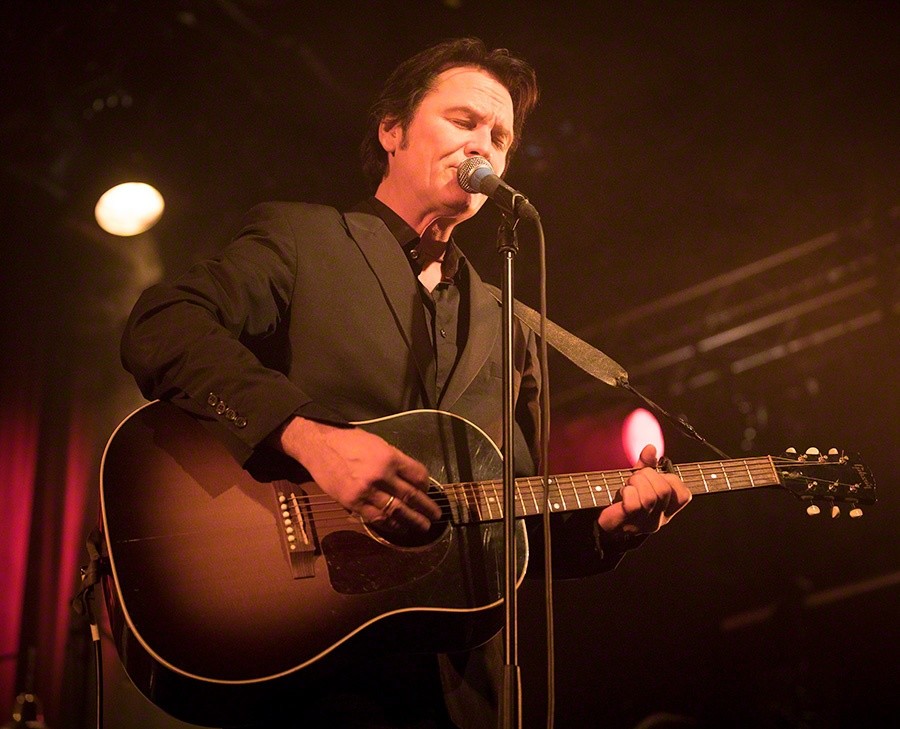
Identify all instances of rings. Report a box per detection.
[381,495,394,512]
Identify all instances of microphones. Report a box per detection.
[457,156,540,224]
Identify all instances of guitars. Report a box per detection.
[93,397,884,728]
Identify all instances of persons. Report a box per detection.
[121,37,691,729]
